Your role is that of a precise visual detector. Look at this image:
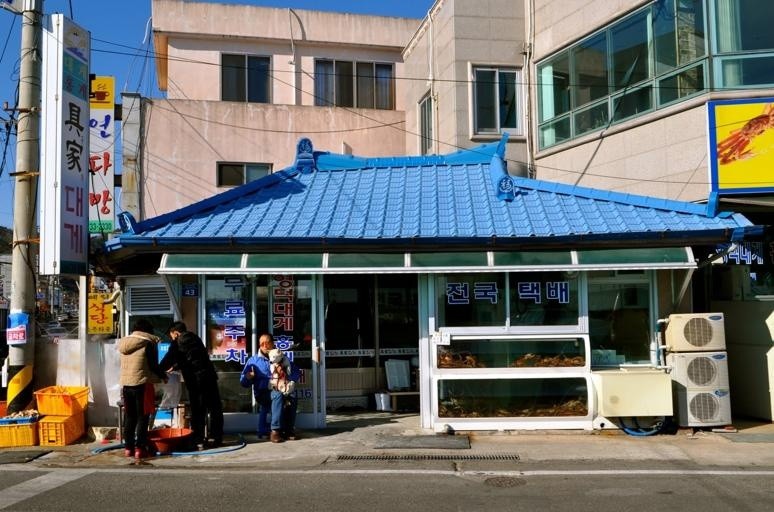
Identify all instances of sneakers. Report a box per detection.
[125,448,156,459]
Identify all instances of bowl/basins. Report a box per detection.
[146,427,194,453]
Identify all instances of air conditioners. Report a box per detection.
[661,310,735,431]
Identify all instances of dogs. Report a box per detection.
[266,347,296,405]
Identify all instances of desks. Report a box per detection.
[115,397,211,446]
[385,387,419,414]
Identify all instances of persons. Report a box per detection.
[118,319,169,459]
[240,334,300,443]
[160,321,224,451]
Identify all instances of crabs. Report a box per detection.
[715,102,774,165]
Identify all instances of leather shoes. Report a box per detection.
[271,431,298,443]
